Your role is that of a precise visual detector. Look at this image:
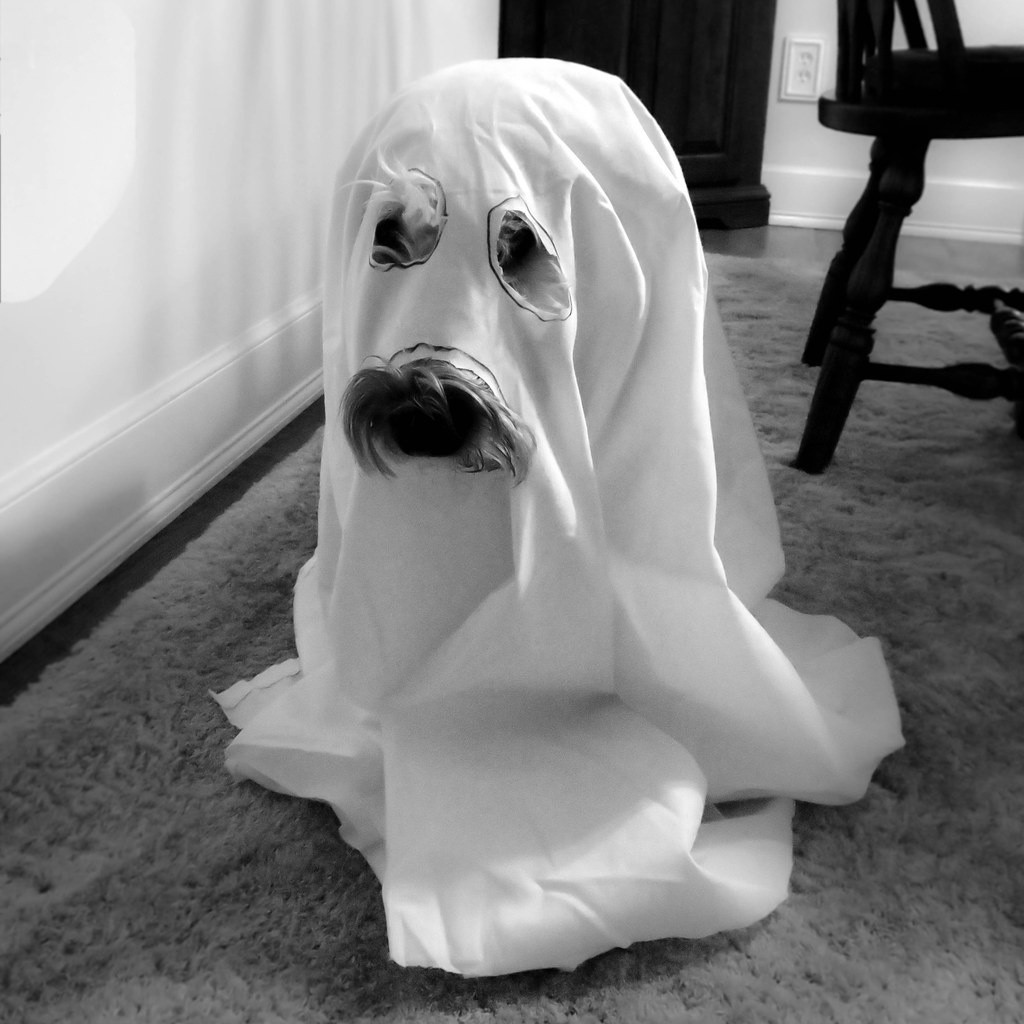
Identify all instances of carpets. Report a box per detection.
[0,253,1024,1024]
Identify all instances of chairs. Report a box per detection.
[791,0,1024,474]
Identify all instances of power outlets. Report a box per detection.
[779,36,824,101]
